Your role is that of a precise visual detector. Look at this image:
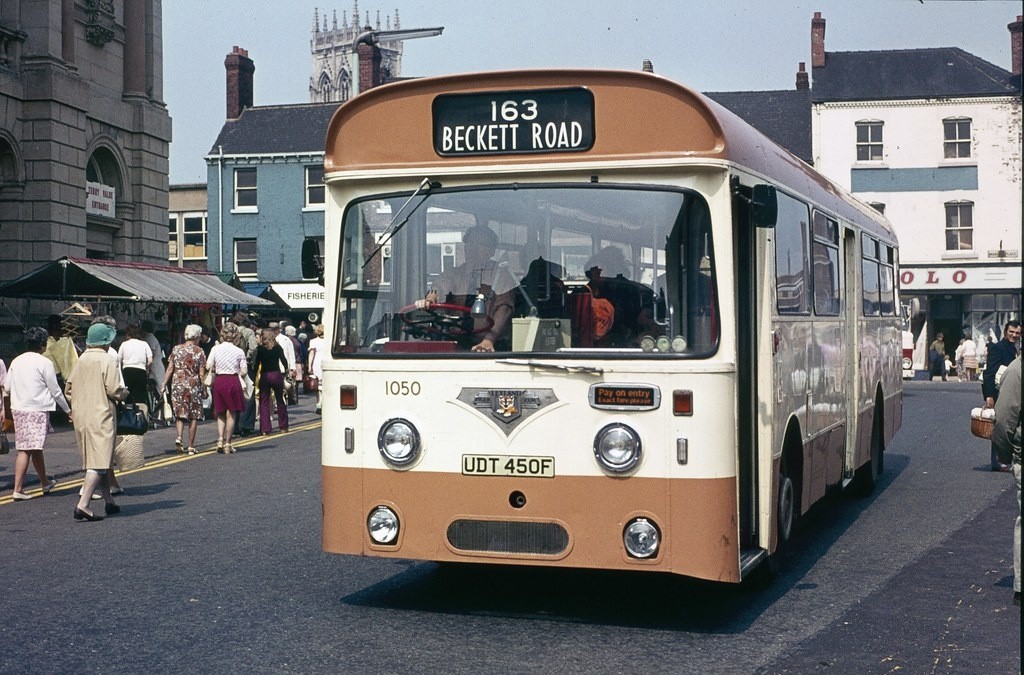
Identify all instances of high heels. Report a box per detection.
[188,447,198,455]
[225,443,236,453]
[74,507,104,521]
[175,436,183,453]
[105,503,120,515]
[217,442,223,452]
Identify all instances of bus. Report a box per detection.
[900,301,916,378]
[301,67,904,584]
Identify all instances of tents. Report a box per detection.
[0,255,324,434]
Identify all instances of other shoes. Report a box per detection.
[233,428,251,436]
[13,492,33,501]
[79,489,103,499]
[316,409,321,414]
[110,486,123,494]
[991,464,1012,472]
[49,424,54,433]
[1013,592,1021,603]
[42,480,56,495]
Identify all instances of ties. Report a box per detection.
[457,270,477,350]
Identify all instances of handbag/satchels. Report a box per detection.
[0,431,9,454]
[204,369,216,387]
[303,372,318,394]
[116,391,148,436]
[163,391,172,420]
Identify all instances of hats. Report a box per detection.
[937,333,944,337]
[86,323,116,348]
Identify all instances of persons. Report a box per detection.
[953,338,967,384]
[252,328,293,439]
[928,333,948,383]
[991,345,1021,604]
[414,224,516,354]
[583,245,651,350]
[195,316,324,429]
[161,323,205,455]
[4,327,76,499]
[63,321,132,521]
[205,321,248,455]
[944,354,957,380]
[136,320,171,430]
[512,239,563,347]
[961,332,978,379]
[983,320,1023,470]
[119,330,153,435]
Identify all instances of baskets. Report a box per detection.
[971,404,996,439]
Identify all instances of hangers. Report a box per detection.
[58,294,225,339]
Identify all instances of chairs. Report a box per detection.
[520,280,643,347]
